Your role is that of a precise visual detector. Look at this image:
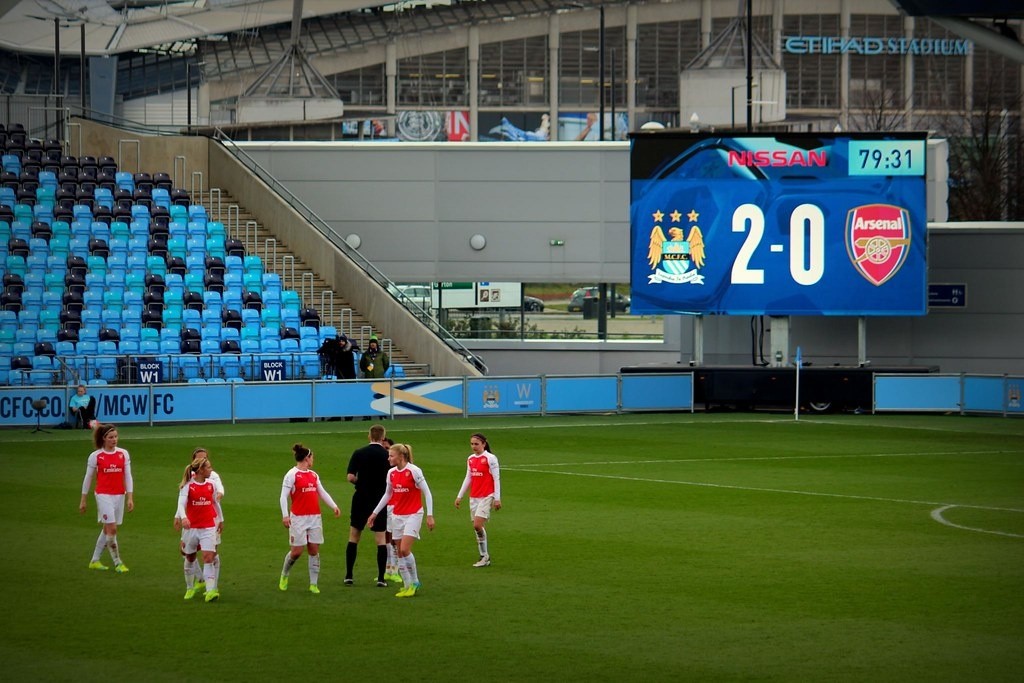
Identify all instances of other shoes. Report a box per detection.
[204,589,219,602]
[88,561,109,570]
[309,584,320,594]
[374,573,391,581]
[115,563,129,573]
[472,555,490,567]
[390,574,402,582]
[278,574,288,591]
[194,582,207,592]
[395,587,408,597]
[343,579,353,585]
[202,589,218,595]
[402,582,421,597]
[476,528,486,544]
[184,589,195,600]
[377,580,388,587]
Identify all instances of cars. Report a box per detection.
[567,286,630,315]
[458,295,544,315]
[387,285,441,318]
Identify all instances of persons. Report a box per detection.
[327,335,356,421]
[360,332,389,420]
[344,425,393,587]
[173,448,225,596]
[575,113,597,140]
[367,443,435,597]
[80,424,133,573]
[178,457,224,602]
[455,434,501,567]
[70,384,96,429]
[279,444,340,593]
[373,438,402,583]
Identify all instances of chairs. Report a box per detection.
[0,122,405,390]
